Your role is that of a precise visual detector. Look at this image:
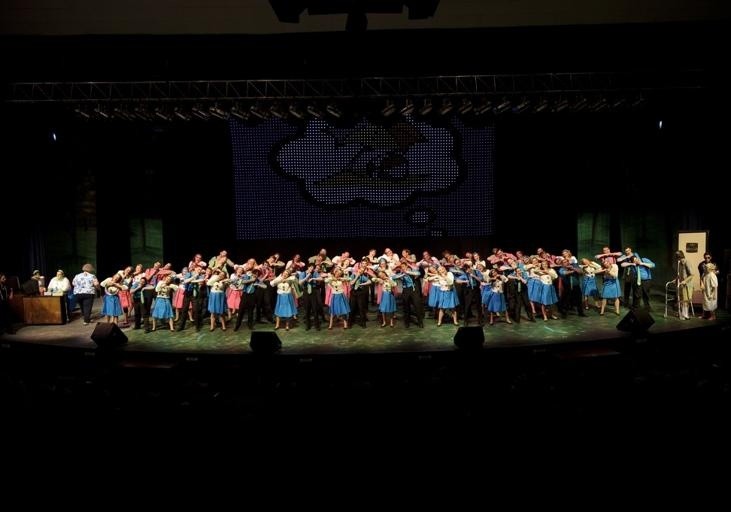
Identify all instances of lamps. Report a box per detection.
[70,87,647,124]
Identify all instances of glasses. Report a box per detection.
[704,257,710,259]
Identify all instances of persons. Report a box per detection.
[1,264,100,326]
[669,250,719,320]
[100,248,654,331]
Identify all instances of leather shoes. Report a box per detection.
[686,316,716,320]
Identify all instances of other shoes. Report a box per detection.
[123,305,620,332]
